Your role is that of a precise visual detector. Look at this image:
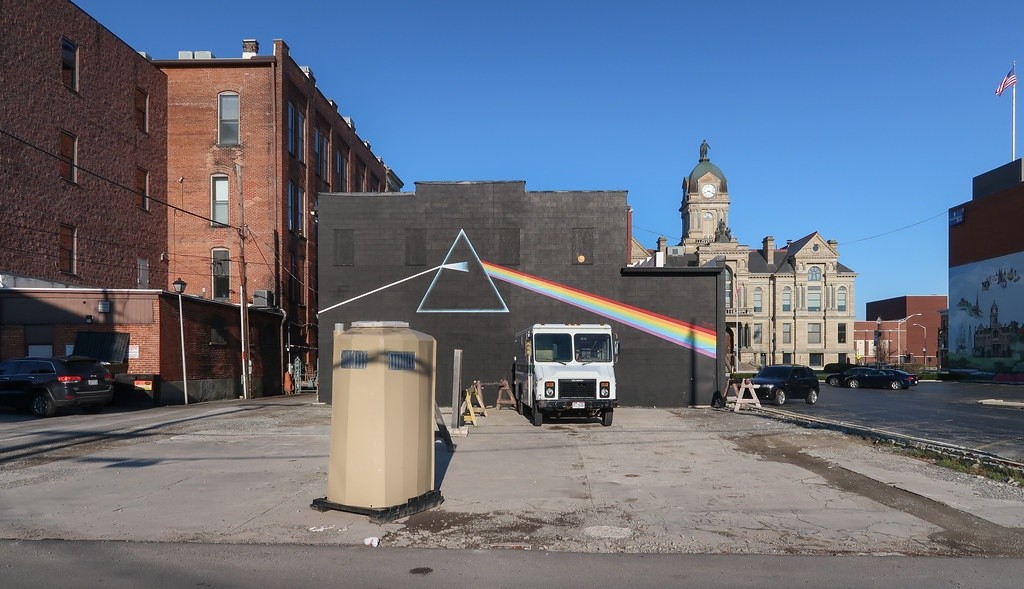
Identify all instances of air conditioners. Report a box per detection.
[253,290,272,308]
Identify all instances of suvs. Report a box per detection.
[739,364,820,406]
[0,355,112,418]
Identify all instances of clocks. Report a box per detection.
[702,184,715,198]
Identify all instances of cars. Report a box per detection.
[824,367,921,389]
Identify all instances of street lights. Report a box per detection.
[913,323,926,370]
[172,277,190,407]
[898,313,922,370]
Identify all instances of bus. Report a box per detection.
[513,323,622,427]
